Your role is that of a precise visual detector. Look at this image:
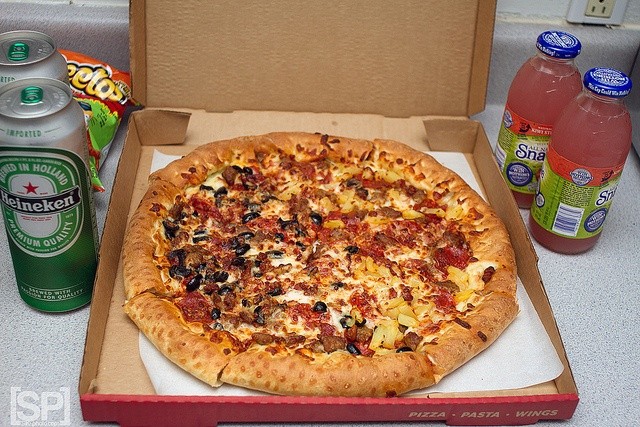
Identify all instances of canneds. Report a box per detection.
[0,29,71,88]
[1,76,100,311]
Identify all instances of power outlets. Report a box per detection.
[566,0,629,27]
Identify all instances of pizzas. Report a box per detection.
[121,132,519,396]
